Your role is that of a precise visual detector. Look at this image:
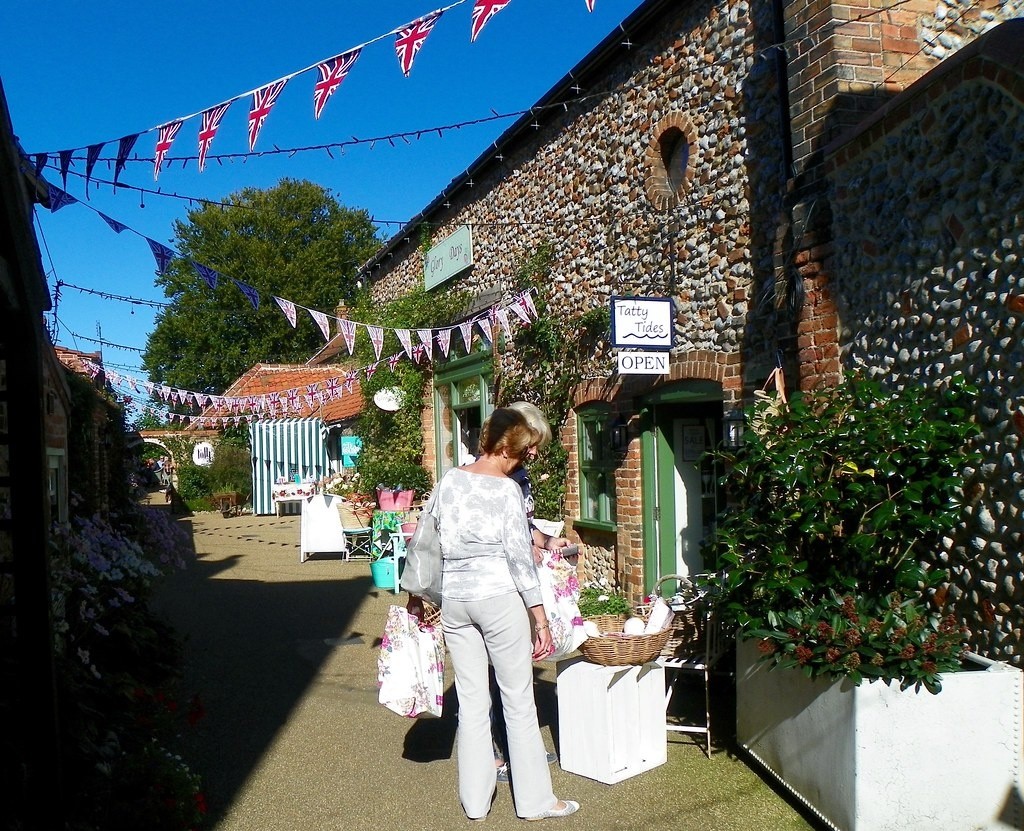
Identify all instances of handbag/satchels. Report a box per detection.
[376,595,444,719]
[531,550,587,658]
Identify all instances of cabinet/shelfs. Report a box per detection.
[656,650,724,759]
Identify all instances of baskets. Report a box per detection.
[581,613,672,667]
[644,574,710,657]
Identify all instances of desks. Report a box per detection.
[391,532,414,594]
[273,483,315,519]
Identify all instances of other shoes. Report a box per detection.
[528,798,581,821]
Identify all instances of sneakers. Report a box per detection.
[495,763,512,783]
[545,750,558,765]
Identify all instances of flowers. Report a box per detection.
[312,473,364,499]
[1,457,212,820]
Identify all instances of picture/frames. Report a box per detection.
[609,295,674,350]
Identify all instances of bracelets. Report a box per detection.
[543,535,552,550]
[533,620,549,631]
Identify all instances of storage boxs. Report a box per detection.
[633,573,709,657]
[577,613,673,667]
[370,562,397,588]
[376,487,414,511]
[336,502,376,530]
[401,523,417,544]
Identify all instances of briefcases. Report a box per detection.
[339,501,374,529]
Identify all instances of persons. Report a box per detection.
[486,401,582,783]
[143,454,172,490]
[407,408,581,820]
